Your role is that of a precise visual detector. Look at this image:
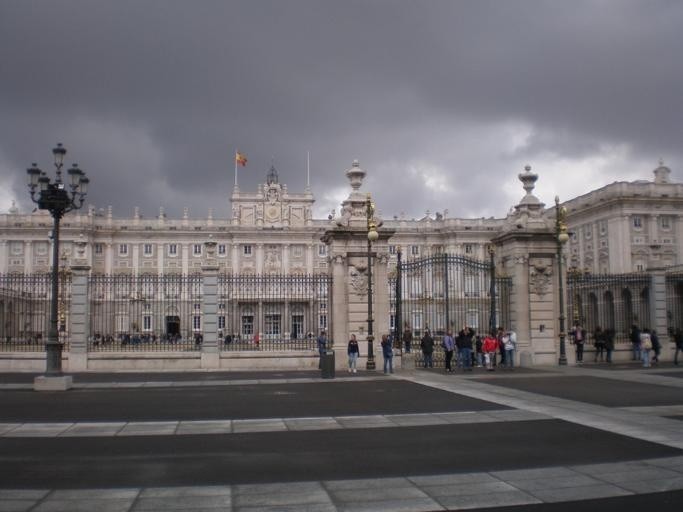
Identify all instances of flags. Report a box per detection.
[236,151,248,167]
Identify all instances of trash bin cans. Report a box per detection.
[322,349,335,379]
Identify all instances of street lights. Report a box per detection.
[364,192,386,371]
[26,138,92,393]
[551,195,575,370]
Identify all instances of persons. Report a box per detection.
[194,334,203,349]
[6,331,41,344]
[121,333,157,345]
[346,334,359,374]
[161,332,182,344]
[672,328,682,367]
[283,330,297,344]
[381,334,395,375]
[317,330,328,368]
[305,331,311,343]
[255,331,259,347]
[403,326,412,355]
[92,334,114,345]
[570,318,663,367]
[421,321,517,375]
[218,331,242,344]
